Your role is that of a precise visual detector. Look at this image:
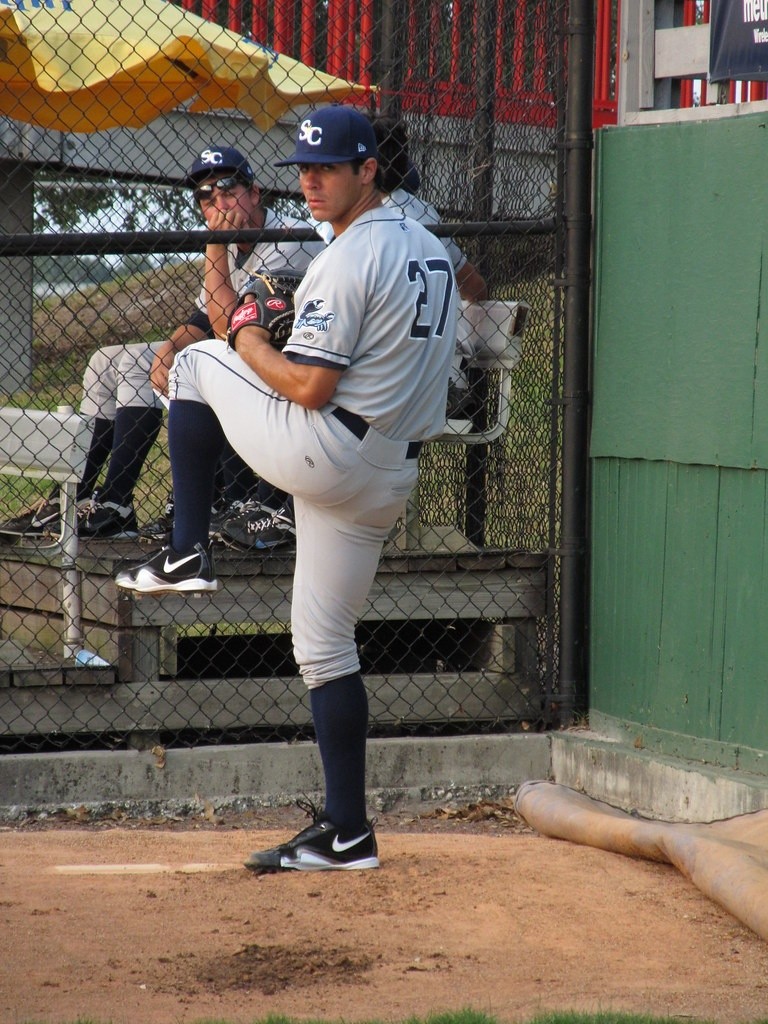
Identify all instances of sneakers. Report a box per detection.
[220,499,296,553]
[0,482,92,536]
[135,488,176,540]
[208,491,281,536]
[112,539,218,592]
[43,485,139,543]
[242,792,380,871]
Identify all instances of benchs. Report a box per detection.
[419,293,531,547]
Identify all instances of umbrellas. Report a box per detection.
[1,0,378,130]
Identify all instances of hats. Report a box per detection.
[273,103,378,167]
[183,145,256,190]
[364,112,420,197]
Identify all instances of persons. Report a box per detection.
[114,102,456,876]
[135,115,486,552]
[0,146,326,543]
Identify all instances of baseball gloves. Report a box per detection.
[226,271,296,353]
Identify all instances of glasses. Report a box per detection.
[193,176,247,205]
